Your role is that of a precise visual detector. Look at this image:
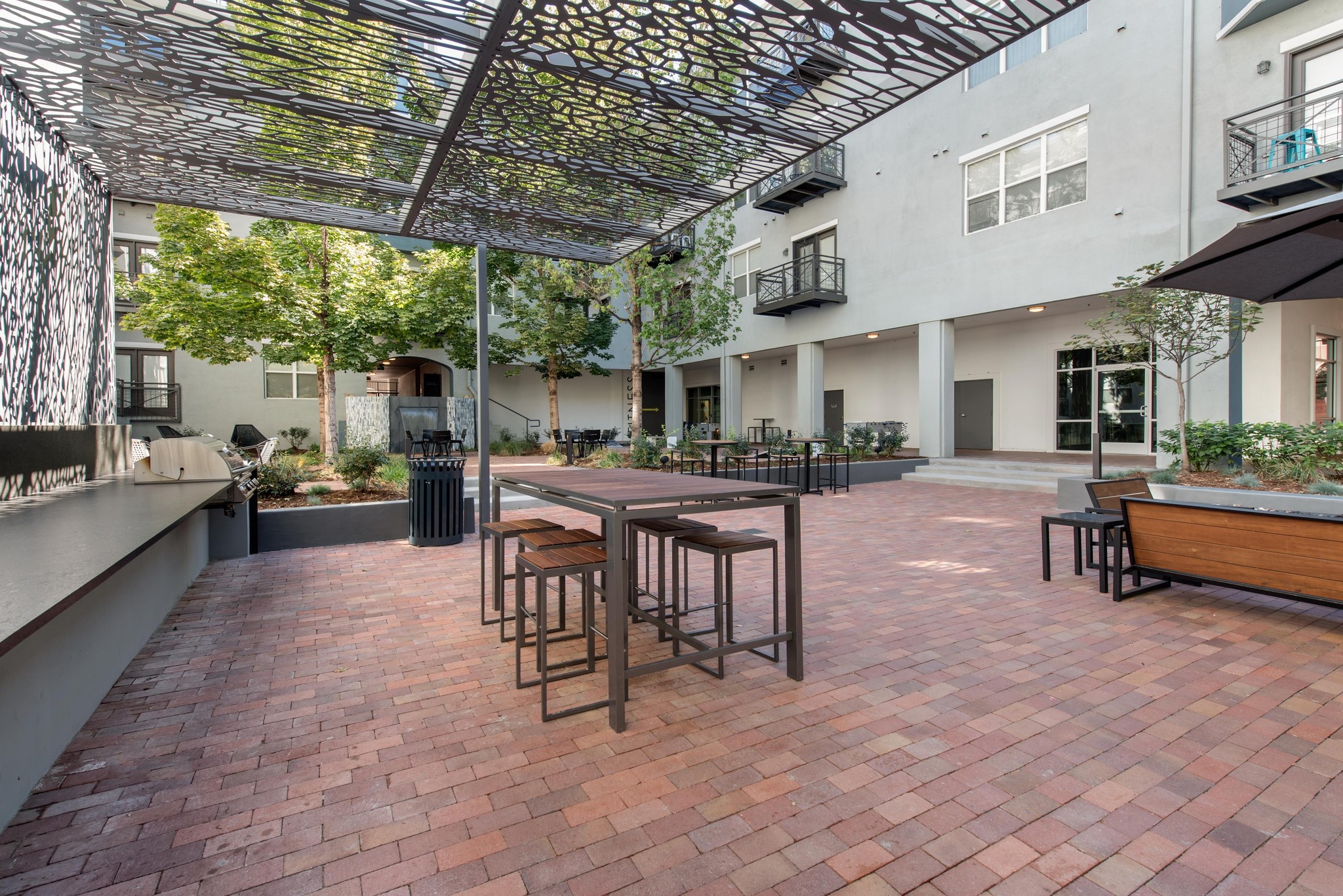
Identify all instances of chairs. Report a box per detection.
[816,445,849,494]
[551,426,613,463]
[403,424,467,459]
[725,448,758,501]
[254,437,278,465]
[1085,477,1154,574]
[671,450,704,506]
[767,447,800,497]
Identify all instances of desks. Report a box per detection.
[1041,512,1124,593]
[785,438,830,496]
[690,440,739,504]
[753,417,775,444]
[491,468,803,732]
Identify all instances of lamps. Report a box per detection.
[1026,306,1046,312]
[866,333,879,339]
[741,354,750,359]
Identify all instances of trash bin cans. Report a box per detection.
[406,455,467,547]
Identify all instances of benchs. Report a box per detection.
[1113,496,1343,611]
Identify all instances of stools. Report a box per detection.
[1267,128,1323,175]
[630,518,719,642]
[516,545,629,723]
[517,529,607,673]
[746,426,763,444]
[478,518,566,643]
[672,530,779,681]
[621,514,672,615]
[669,233,691,251]
[764,427,782,444]
